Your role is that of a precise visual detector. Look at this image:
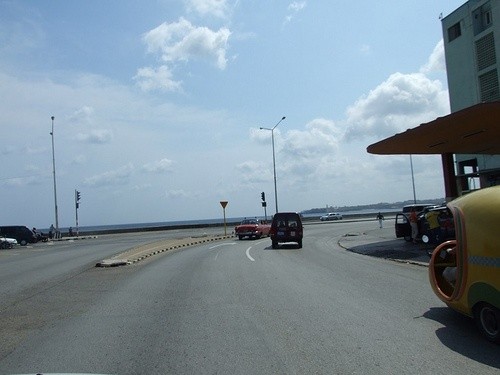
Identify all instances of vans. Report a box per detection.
[270,212,303,249]
[0,225,38,246]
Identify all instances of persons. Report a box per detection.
[442,246,456,288]
[409,207,419,244]
[376,212,384,228]
[423,207,440,245]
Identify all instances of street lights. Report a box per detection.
[259,116,286,213]
[50,115,59,238]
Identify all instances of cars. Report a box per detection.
[0,235,18,249]
[234,217,272,240]
[319,212,344,221]
[394,203,447,247]
[428,185,500,348]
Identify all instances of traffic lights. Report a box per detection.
[261,192,265,201]
[262,202,266,207]
[77,190,80,201]
